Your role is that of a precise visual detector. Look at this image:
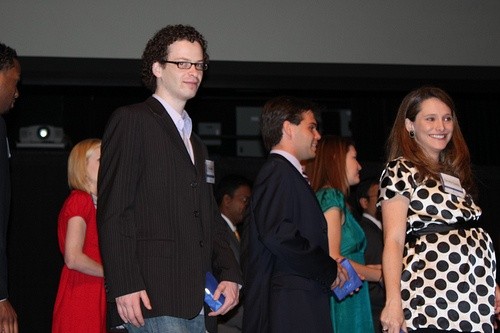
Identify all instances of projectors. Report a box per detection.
[19,124,64,143]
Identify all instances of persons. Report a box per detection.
[375,87,500,333]
[51,139,107,333]
[98,24,242,333]
[356,183,386,333]
[0,43,19,333]
[302,135,384,333]
[239,96,362,333]
[213,173,251,332]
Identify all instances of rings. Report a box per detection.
[339,272,343,273]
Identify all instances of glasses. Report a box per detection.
[162,60,208,70]
